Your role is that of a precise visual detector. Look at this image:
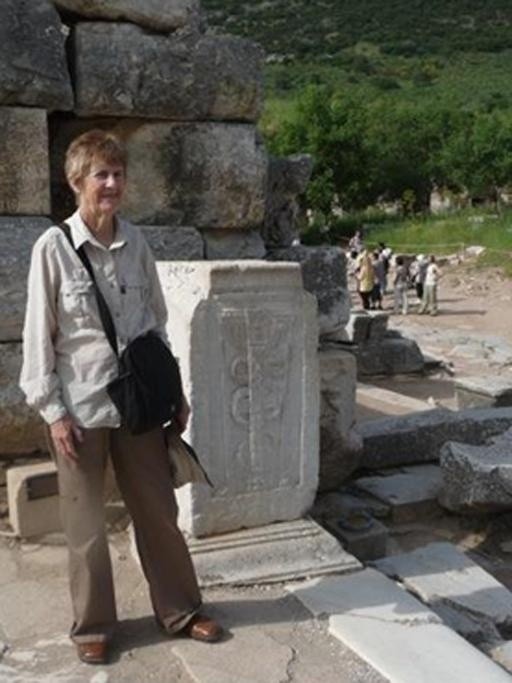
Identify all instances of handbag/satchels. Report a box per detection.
[106,331,180,435]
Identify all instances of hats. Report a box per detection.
[166,429,214,490]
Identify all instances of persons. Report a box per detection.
[19,128,226,664]
[344,227,442,316]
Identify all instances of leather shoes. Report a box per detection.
[181,613,223,642]
[78,642,110,664]
